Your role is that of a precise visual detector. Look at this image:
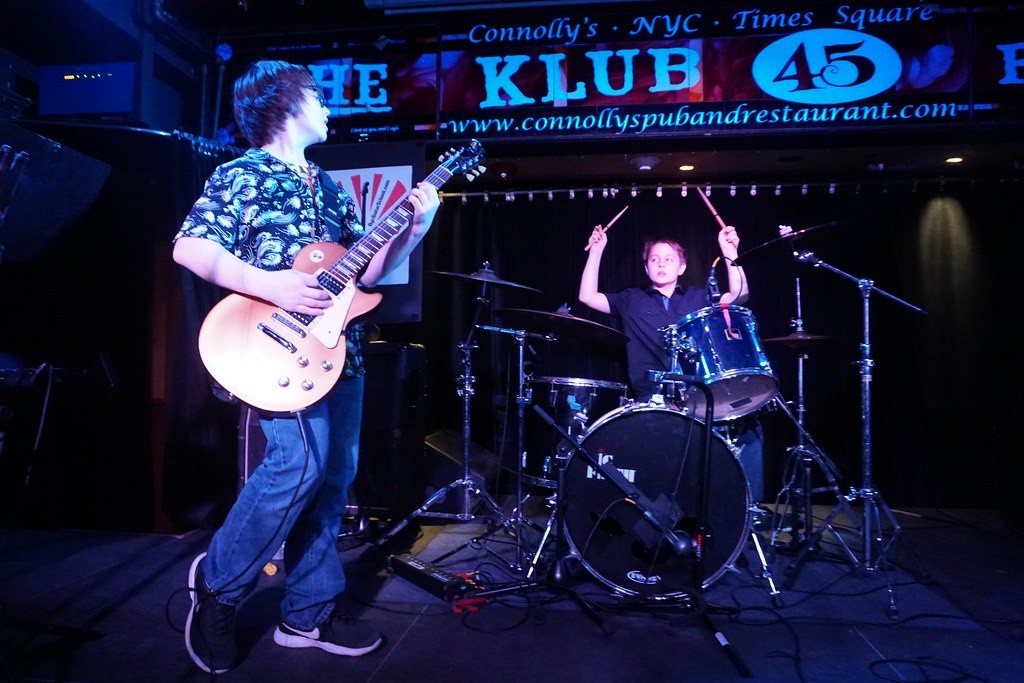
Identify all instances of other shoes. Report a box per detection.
[751,502,773,528]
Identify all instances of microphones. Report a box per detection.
[703,267,716,301]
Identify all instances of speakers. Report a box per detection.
[229,342,424,523]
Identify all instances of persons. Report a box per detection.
[172,59,440,674]
[579,224,777,564]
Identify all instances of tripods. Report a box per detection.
[375,297,575,578]
[766,273,930,586]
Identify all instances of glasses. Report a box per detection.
[300,85,326,107]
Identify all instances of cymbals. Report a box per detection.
[507,305,632,343]
[728,217,851,268]
[428,268,542,298]
[766,333,839,343]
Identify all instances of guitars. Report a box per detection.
[196,139,488,415]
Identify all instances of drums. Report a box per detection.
[517,373,629,492]
[663,302,778,422]
[552,402,751,602]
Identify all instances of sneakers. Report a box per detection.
[274,613,383,657]
[165,552,238,675]
